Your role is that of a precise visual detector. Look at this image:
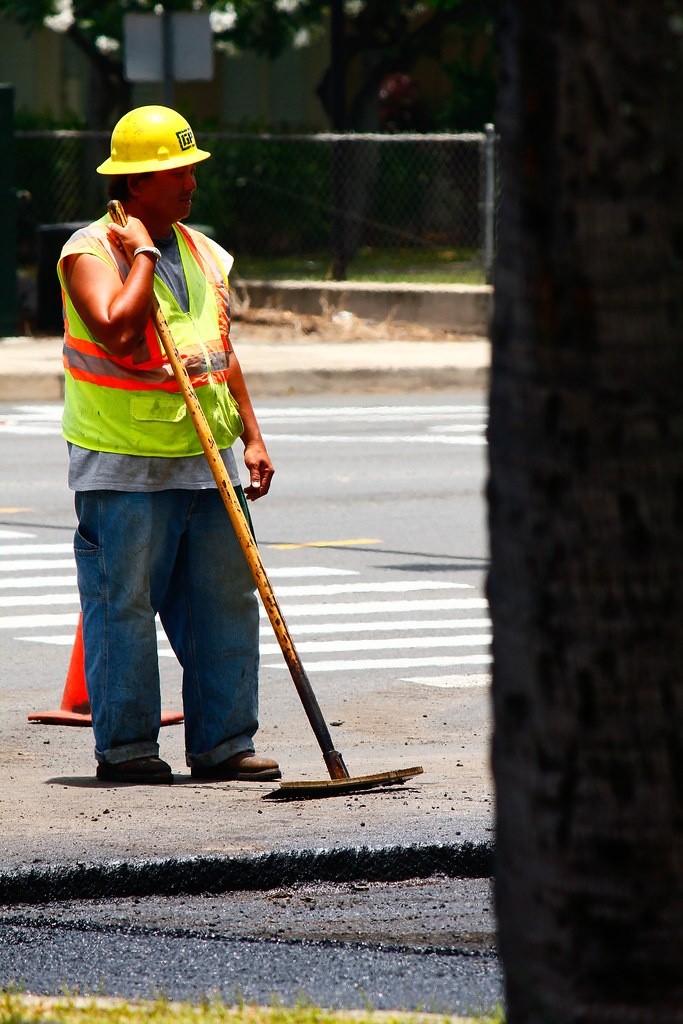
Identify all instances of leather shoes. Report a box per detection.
[96,754,173,783]
[191,752,279,781]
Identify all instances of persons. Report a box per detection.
[56,106,281,781]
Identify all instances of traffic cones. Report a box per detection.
[27,609,184,728]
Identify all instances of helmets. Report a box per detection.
[96,104,211,175]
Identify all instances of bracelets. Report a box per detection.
[135,246,162,262]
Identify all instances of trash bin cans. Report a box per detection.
[33,220,94,331]
